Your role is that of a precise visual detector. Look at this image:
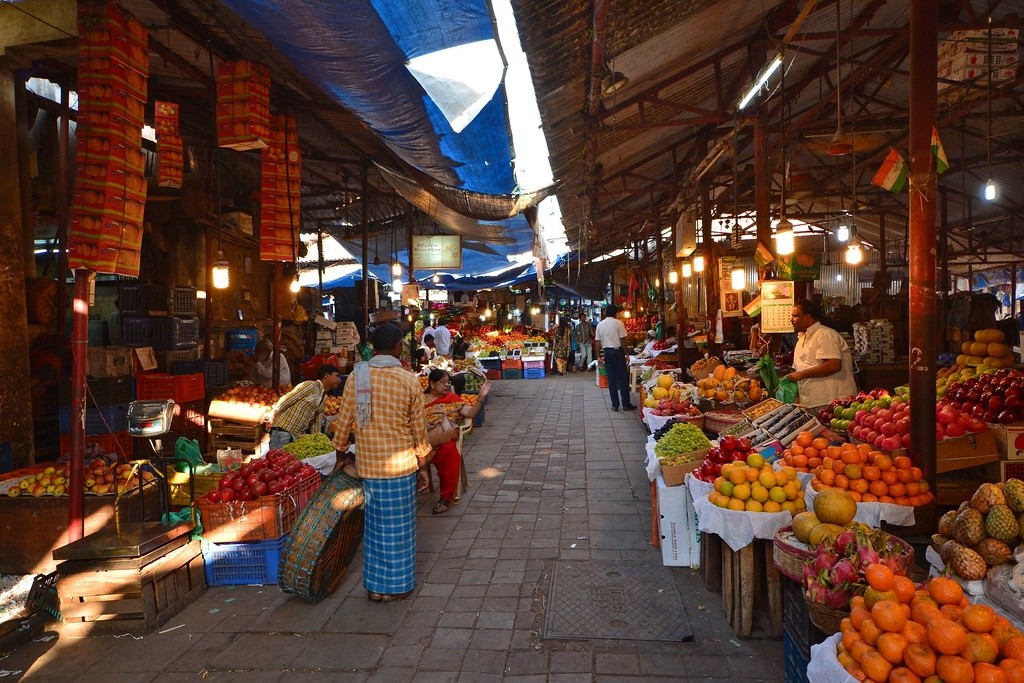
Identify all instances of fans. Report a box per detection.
[797,0,890,157]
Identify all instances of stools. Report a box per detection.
[630,366,643,393]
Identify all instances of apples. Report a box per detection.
[8,459,154,496]
[692,434,758,484]
[819,364,986,450]
[216,384,342,415]
[941,368,1024,425]
[446,321,526,346]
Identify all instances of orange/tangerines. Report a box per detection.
[836,562,1024,683]
[469,335,547,351]
[459,393,478,405]
[709,431,935,513]
[418,376,429,390]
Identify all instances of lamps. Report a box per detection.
[599,61,630,97]
[738,49,787,111]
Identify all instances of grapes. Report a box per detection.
[282,432,335,460]
[654,422,713,458]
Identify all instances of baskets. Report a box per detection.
[644,407,744,433]
[774,524,914,584]
[802,586,860,635]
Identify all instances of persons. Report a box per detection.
[699,345,708,358]
[415,316,468,373]
[595,304,637,410]
[643,330,657,354]
[774,299,857,415]
[332,324,432,604]
[423,369,491,513]
[554,314,594,375]
[752,323,761,358]
[271,364,341,448]
[240,340,291,388]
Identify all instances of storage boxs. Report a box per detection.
[314,314,360,352]
[649,398,1024,683]
[58,283,228,462]
[460,360,545,427]
[191,470,321,587]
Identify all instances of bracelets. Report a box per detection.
[477,399,483,403]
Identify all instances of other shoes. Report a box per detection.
[623,404,637,410]
[383,590,413,600]
[369,591,383,600]
[611,406,618,411]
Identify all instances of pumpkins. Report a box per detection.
[956,329,1016,369]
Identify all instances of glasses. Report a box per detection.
[436,380,451,385]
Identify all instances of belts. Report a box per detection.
[271,426,285,431]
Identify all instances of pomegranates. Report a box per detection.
[204,447,315,502]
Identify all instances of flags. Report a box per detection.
[642,270,658,301]
[754,242,776,267]
[872,150,908,192]
[931,128,949,173]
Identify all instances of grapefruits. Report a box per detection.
[791,488,870,547]
[643,376,681,407]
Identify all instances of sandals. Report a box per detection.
[432,502,448,512]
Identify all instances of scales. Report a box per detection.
[51,399,195,569]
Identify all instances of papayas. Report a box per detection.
[695,365,763,402]
